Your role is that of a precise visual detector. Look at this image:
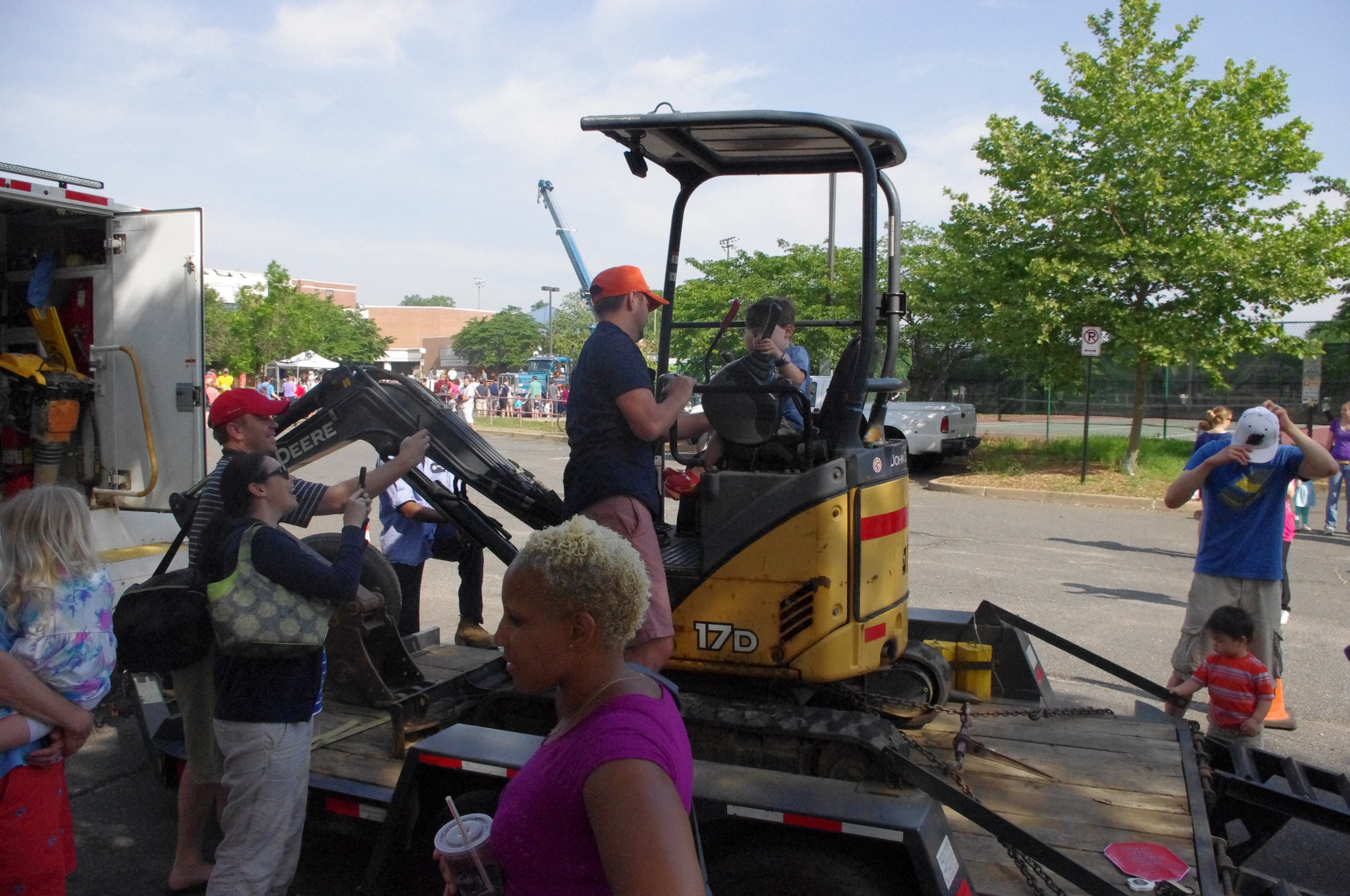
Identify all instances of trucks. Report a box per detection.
[0,157,210,527]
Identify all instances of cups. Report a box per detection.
[435,813,506,896]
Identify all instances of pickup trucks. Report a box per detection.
[690,376,983,474]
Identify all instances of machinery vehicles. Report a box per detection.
[156,107,956,794]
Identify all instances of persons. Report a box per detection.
[0,568,96,896]
[662,296,813,494]
[376,442,505,650]
[201,348,573,426]
[1189,398,1350,617]
[1159,394,1341,720]
[431,513,709,895]
[160,383,435,896]
[203,451,374,896]
[0,484,118,755]
[562,266,711,677]
[1161,605,1276,749]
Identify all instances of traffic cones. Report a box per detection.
[1265,675,1296,728]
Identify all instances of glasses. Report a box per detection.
[256,463,291,483]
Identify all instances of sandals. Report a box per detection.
[661,467,701,500]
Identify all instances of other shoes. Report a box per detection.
[1294,524,1350,535]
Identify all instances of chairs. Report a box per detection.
[799,334,884,459]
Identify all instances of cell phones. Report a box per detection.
[356,466,367,499]
[416,413,422,437]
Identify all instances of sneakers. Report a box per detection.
[455,614,501,649]
[1279,608,1290,625]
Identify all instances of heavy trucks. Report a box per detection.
[495,356,573,416]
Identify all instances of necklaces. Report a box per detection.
[550,673,646,736]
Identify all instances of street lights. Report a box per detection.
[541,284,560,358]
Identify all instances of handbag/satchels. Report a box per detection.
[204,521,331,657]
[113,566,208,674]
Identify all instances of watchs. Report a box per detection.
[774,353,791,368]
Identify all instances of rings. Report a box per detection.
[56,757,59,762]
[1276,405,1279,410]
[364,501,369,507]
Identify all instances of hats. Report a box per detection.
[1231,406,1280,463]
[589,265,669,313]
[208,389,291,430]
